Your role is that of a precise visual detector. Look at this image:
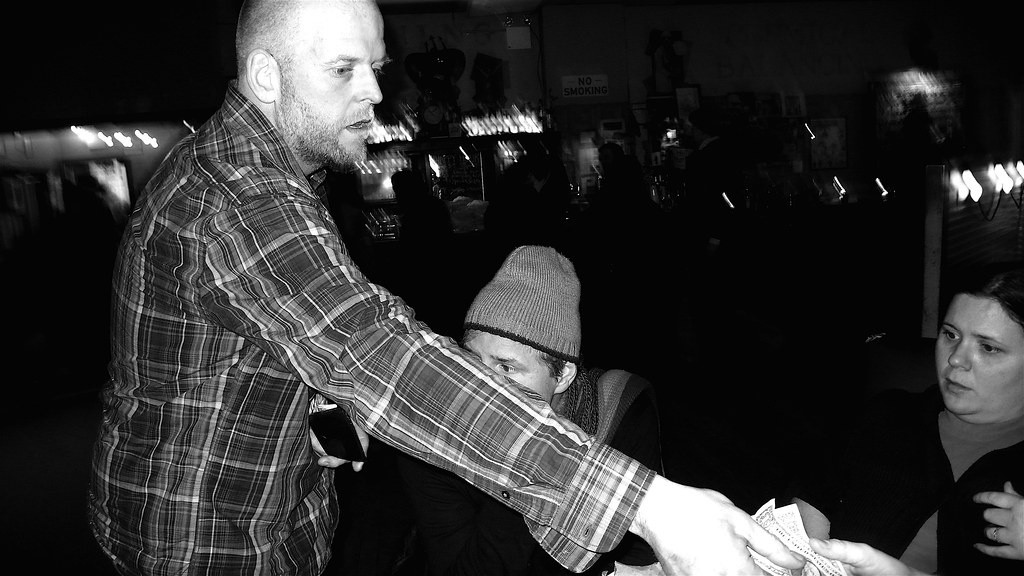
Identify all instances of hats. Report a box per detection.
[464,245,582,364]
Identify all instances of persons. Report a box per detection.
[396,245,668,576]
[802,269,1024,576]
[597,144,642,196]
[84,1,800,576]
[392,172,449,279]
[683,108,735,360]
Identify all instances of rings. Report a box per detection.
[994,527,1002,542]
